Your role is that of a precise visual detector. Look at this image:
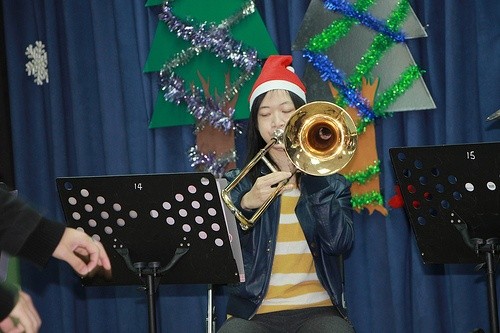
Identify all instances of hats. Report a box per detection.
[248,55,307,112]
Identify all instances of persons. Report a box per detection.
[218,56,356,333]
[0,181,112,333]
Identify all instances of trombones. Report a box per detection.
[221,101,358,228]
